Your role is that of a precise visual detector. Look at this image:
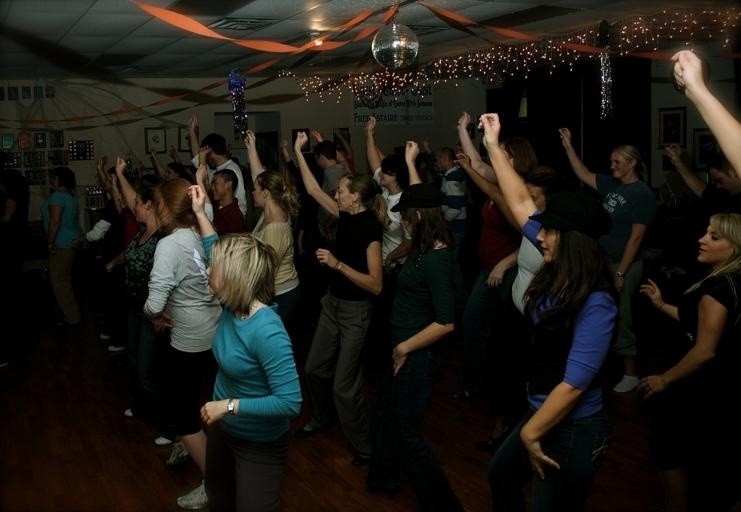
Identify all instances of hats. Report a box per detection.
[390,183,442,212]
[529,198,613,239]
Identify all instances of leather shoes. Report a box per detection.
[296,416,338,438]
[479,425,508,450]
[352,453,402,499]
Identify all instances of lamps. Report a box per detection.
[370,3,419,71]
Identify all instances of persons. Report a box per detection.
[1,112,741,511]
[669,51,741,180]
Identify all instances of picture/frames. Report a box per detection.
[176,122,200,152]
[142,124,167,154]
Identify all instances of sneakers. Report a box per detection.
[99,330,126,352]
[613,373,640,392]
[176,477,209,510]
[154,434,189,467]
[58,317,80,331]
[123,408,137,417]
